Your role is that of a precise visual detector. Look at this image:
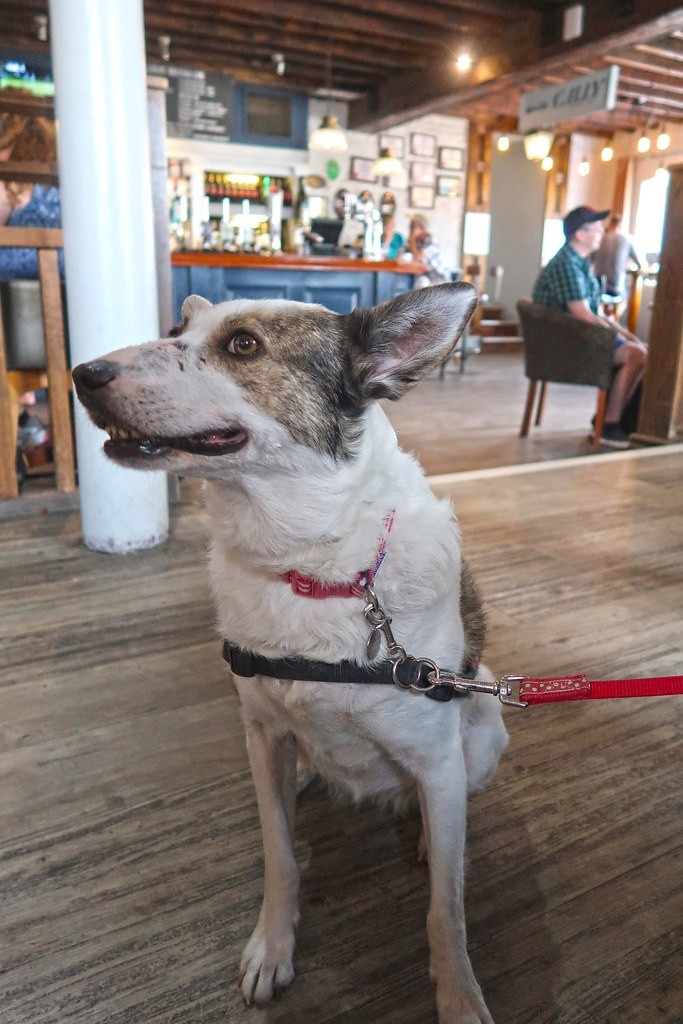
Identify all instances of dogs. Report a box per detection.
[65,275,515,1023]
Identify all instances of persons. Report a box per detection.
[531,204,649,450]
[1,86,69,454]
[589,214,645,317]
[376,213,455,288]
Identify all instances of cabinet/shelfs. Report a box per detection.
[169,252,427,327]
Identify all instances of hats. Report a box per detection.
[563,206,610,237]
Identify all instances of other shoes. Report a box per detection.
[16,440,53,477]
[591,422,631,449]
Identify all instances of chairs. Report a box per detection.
[516,298,617,450]
